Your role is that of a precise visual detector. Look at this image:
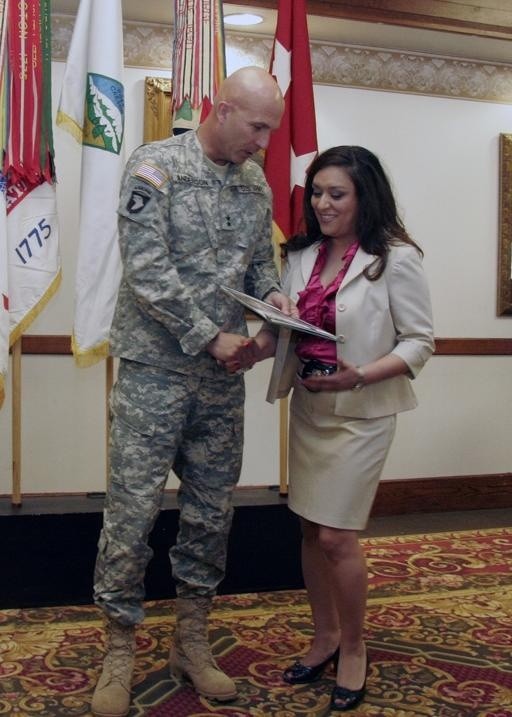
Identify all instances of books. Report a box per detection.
[220,283,337,341]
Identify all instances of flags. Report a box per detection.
[167,1,227,137]
[49,0,130,367]
[261,0,318,250]
[0,0,66,415]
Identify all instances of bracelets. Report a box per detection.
[351,363,366,392]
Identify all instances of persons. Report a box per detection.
[217,144,440,711]
[88,63,300,714]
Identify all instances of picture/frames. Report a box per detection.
[496,132,512,318]
[141,73,201,148]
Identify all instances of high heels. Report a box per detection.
[285,642,369,712]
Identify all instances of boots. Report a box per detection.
[88,617,138,717]
[167,595,239,702]
